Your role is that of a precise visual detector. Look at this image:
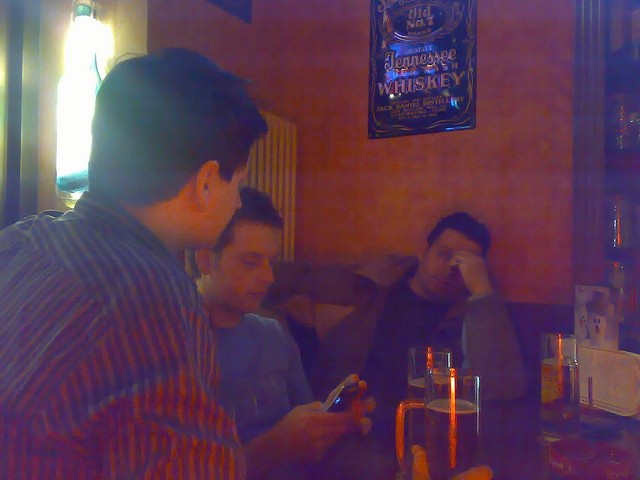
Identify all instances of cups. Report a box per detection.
[405,350,454,468]
[394,367,480,476]
[537,333,580,443]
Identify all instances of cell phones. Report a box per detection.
[321,373,361,412]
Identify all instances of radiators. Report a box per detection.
[182,109,299,280]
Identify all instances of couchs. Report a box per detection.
[260,247,595,404]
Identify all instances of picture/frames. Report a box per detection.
[365,0,479,140]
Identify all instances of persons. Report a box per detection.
[0,46,270,480]
[307,213,528,403]
[190,186,376,480]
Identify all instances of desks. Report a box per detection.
[290,376,636,479]
